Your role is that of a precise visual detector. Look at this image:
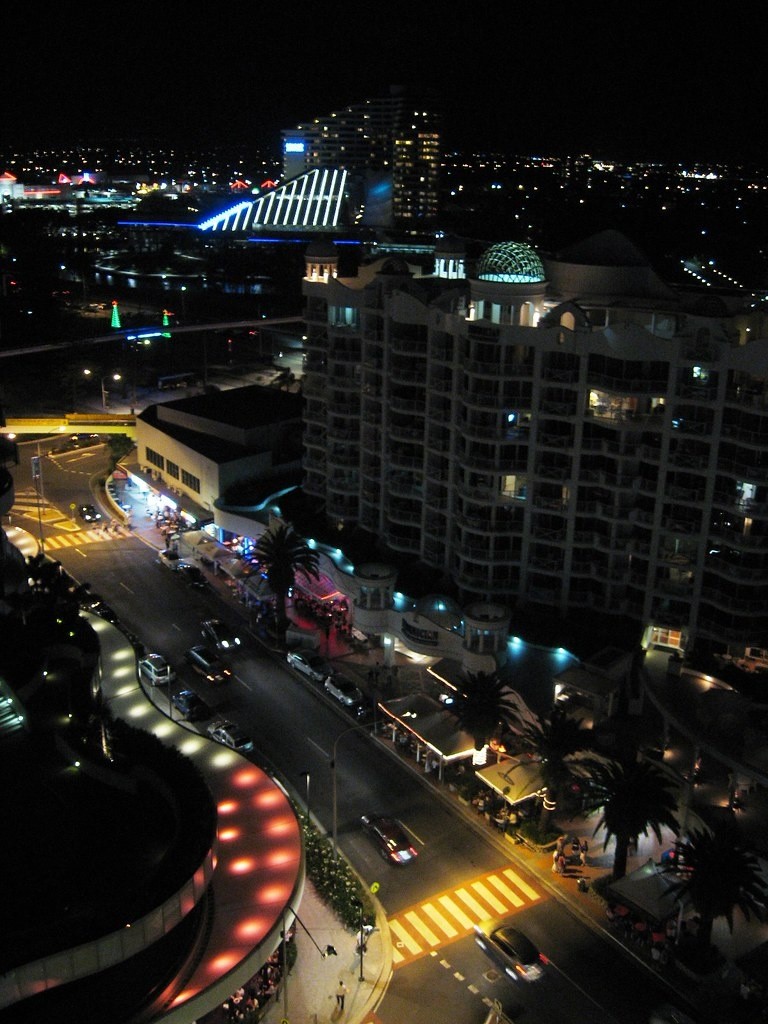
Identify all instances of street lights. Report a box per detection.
[347,897,364,984]
[84,368,121,410]
[31,447,60,555]
[331,710,418,854]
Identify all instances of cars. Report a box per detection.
[285,647,334,683]
[176,563,208,592]
[67,432,99,443]
[158,548,182,573]
[202,614,240,654]
[186,644,233,687]
[78,503,101,524]
[172,690,210,723]
[325,671,363,707]
[207,720,255,757]
[473,916,552,991]
[137,652,177,687]
[358,810,419,868]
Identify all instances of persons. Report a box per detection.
[472,787,526,831]
[336,981,349,1011]
[198,929,291,1024]
[358,930,367,956]
[552,835,589,877]
[295,594,349,633]
[606,902,679,954]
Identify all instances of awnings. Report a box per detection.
[474,753,550,818]
[378,692,489,784]
[606,857,698,922]
